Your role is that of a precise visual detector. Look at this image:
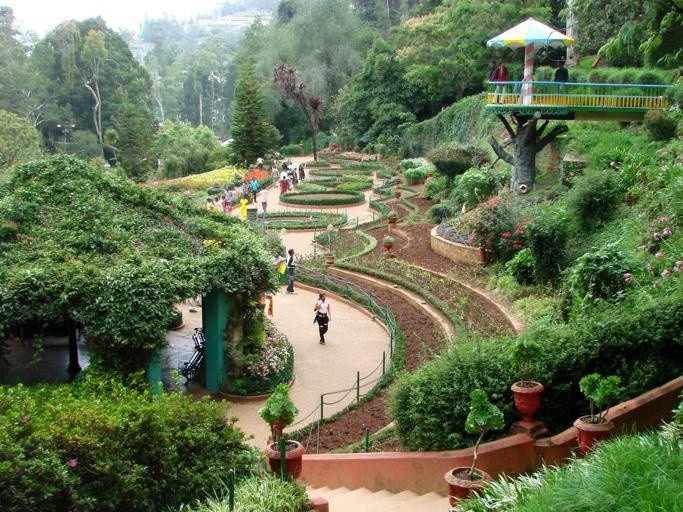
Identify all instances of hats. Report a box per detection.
[318,292,325,300]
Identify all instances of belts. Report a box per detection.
[319,314,327,317]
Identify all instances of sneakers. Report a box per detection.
[319,335,325,344]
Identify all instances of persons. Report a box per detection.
[312,292,331,344]
[205,175,259,218]
[257,187,268,212]
[271,158,305,194]
[553,62,568,84]
[487,63,510,105]
[254,157,264,168]
[269,252,286,269]
[487,60,496,79]
[284,248,295,292]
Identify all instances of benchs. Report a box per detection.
[181,328,207,381]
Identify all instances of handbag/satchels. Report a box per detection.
[313,317,316,324]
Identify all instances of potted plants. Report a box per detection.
[574,370,627,453]
[387,209,397,224]
[322,252,334,263]
[508,334,545,417]
[444,389,507,506]
[258,381,304,478]
[382,235,394,249]
